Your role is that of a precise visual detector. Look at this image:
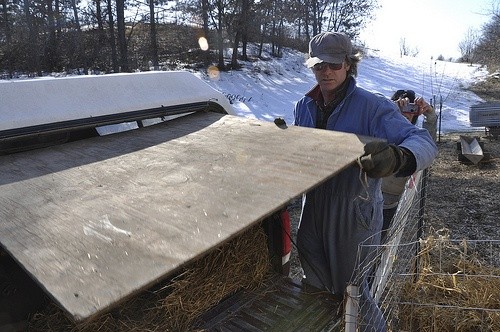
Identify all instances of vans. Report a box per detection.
[0,68,293,331]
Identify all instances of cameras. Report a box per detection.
[401,102,419,114]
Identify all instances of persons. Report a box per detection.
[274,33,438,332]
[382,90,437,264]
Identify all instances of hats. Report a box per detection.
[306,32,352,69]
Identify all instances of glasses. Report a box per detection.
[396,90,416,96]
[312,60,349,71]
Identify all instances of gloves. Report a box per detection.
[358,141,409,178]
